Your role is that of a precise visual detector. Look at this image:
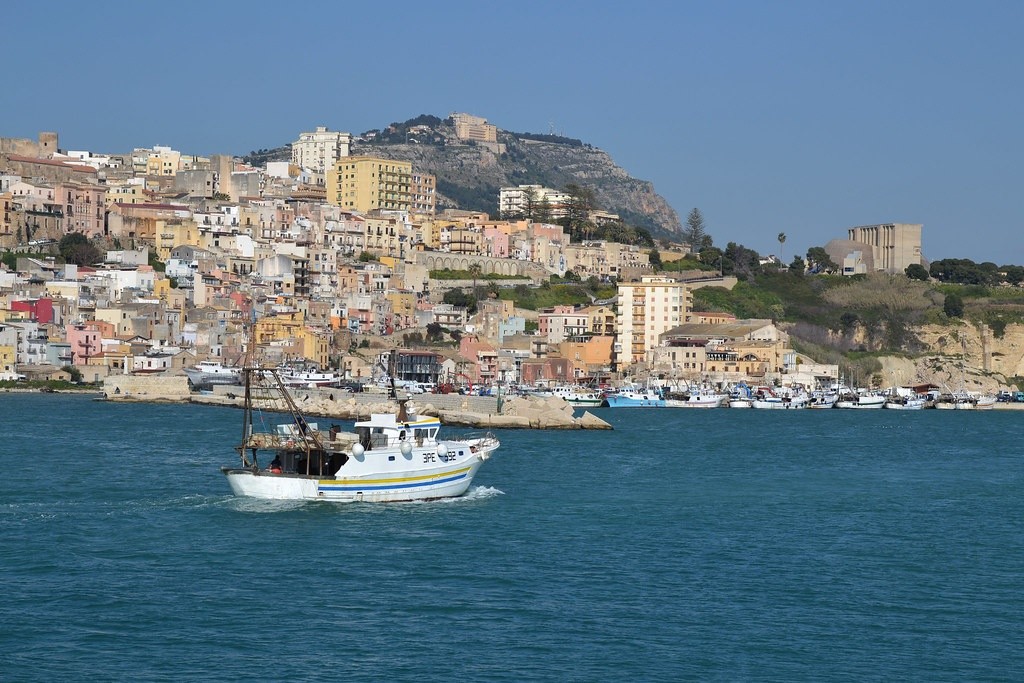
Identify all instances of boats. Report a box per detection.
[462,356,998,411]
[218,365,503,503]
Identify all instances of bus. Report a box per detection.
[414,383,438,394]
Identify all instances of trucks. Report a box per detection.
[998,390,1011,402]
[1012,391,1024,402]
